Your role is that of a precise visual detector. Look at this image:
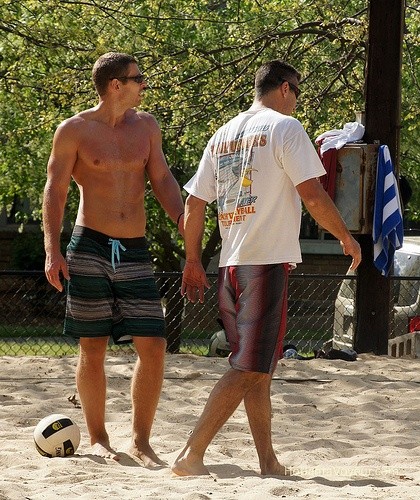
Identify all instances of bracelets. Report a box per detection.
[175,212,185,229]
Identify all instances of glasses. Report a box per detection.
[109,74,144,84]
[278,79,301,98]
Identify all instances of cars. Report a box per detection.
[392,236,420,339]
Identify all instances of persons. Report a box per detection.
[41,51,187,470]
[171,58,364,480]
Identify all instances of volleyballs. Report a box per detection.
[35,414,80,459]
